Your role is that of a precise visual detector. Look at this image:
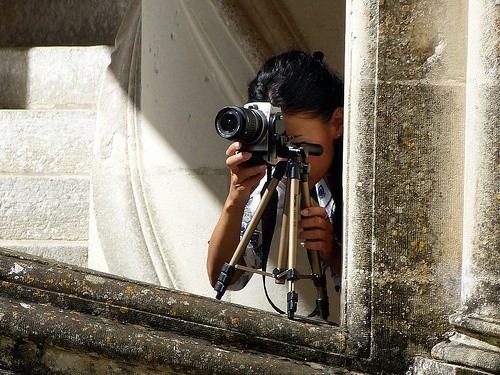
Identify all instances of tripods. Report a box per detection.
[214,141,333,323]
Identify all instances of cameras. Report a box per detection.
[214,102,286,167]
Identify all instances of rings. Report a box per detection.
[320,207,328,219]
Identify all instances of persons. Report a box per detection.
[206,48,343,329]
